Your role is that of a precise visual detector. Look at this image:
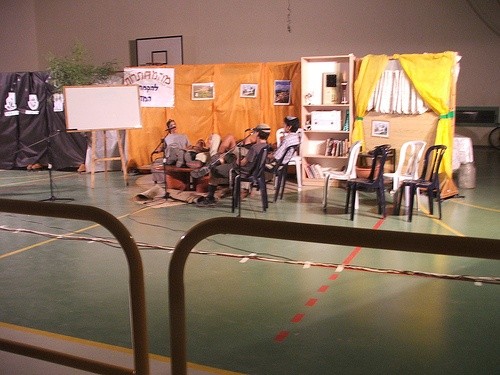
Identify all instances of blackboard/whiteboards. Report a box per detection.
[63,85,144,132]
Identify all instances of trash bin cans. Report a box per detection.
[459,163,476,188]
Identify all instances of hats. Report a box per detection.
[256,124,271,129]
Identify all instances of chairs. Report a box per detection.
[229,127,302,213]
[321,141,447,223]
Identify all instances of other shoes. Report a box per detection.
[197,197,217,208]
[191,166,209,178]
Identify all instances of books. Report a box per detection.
[304,110,351,179]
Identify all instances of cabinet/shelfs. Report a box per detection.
[300,53,354,186]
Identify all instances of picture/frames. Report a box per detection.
[192,83,215,100]
[240,84,257,97]
[274,80,292,105]
[371,120,390,139]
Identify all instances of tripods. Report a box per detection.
[151,130,188,205]
[15,131,75,201]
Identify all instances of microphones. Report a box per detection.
[165,127,176,131]
[55,129,77,131]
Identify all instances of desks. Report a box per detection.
[165,165,209,193]
[452,133,473,171]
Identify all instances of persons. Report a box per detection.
[162,117,298,201]
